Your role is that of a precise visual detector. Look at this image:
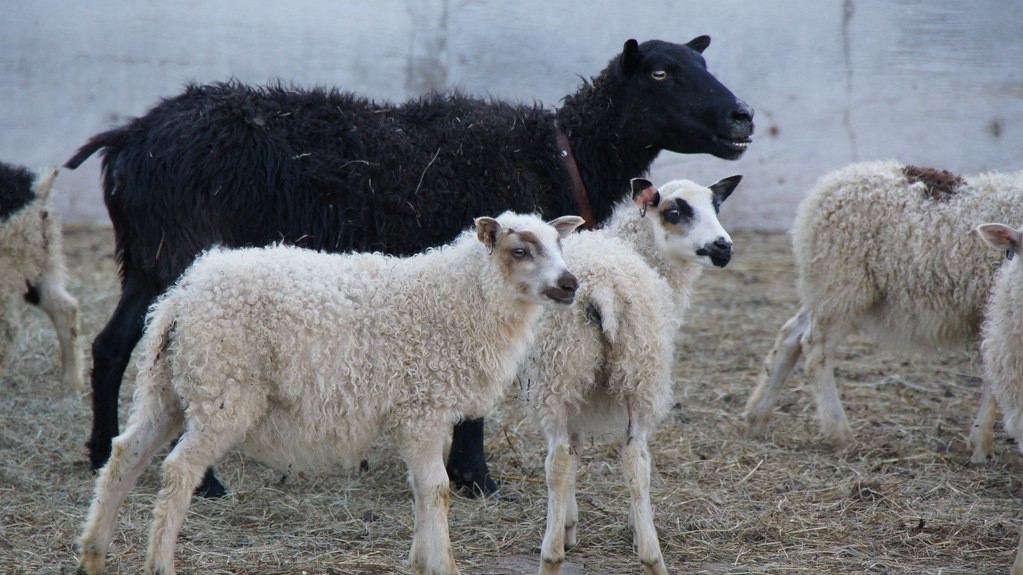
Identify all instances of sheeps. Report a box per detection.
[0,35,1023,575]
